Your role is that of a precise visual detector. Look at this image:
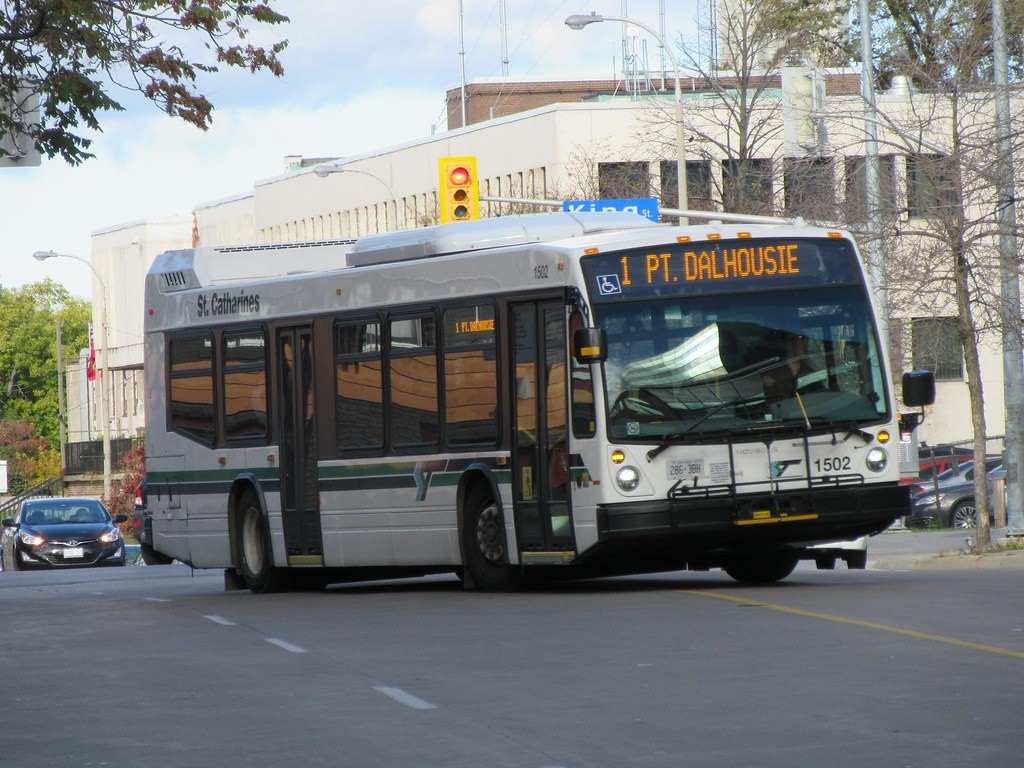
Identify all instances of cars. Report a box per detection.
[892,445,1008,529]
[3,498,129,571]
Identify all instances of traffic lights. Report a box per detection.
[438,154,481,223]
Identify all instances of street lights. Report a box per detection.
[32,249,112,508]
[564,9,690,227]
[312,163,403,231]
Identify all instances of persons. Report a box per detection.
[761,317,826,418]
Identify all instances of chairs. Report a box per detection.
[75,508,89,522]
[742,343,783,419]
[640,370,708,421]
[31,512,45,525]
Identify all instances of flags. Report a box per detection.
[87,325,97,381]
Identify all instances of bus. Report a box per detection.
[142,209,936,592]
